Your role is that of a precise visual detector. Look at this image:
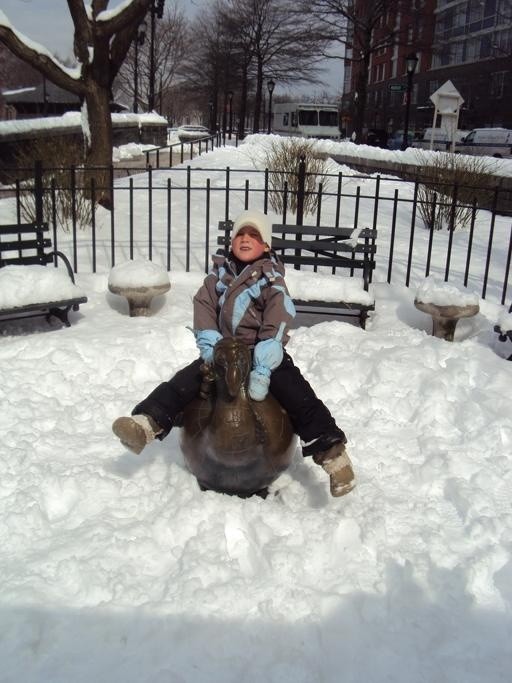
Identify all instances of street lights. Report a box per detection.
[400,47,418,151]
[267,77,275,134]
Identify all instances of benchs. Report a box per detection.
[212,221,379,331]
[0,221,87,337]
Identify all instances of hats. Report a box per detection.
[231,211,271,249]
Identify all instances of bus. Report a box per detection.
[271,102,344,141]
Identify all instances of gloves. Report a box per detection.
[248,370,270,401]
[204,355,215,368]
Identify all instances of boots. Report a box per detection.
[322,451,357,497]
[112,414,155,455]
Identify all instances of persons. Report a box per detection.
[112,210,357,498]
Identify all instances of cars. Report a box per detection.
[419,128,512,159]
